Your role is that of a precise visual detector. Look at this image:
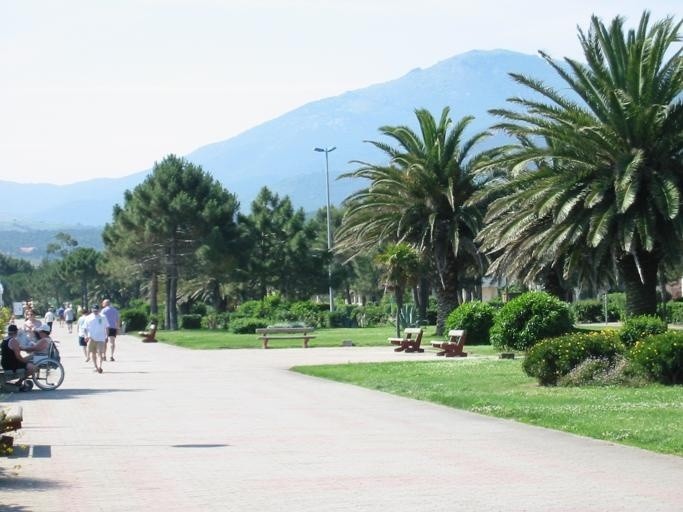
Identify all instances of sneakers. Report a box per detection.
[93,367,103,373]
[84,355,115,362]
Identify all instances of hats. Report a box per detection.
[7,325,20,334]
[39,324,51,333]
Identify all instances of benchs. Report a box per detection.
[136,320,158,342]
[430,328,468,358]
[386,327,424,354]
[0,363,26,396]
[0,403,24,456]
[253,324,315,351]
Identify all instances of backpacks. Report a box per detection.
[48,341,60,369]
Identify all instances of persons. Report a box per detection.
[1,299,120,386]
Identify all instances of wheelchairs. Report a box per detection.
[22,338,64,390]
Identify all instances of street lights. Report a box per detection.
[312,145,339,314]
